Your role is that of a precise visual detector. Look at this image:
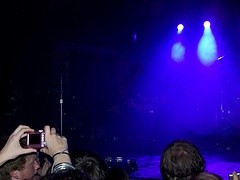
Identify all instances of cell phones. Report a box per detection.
[229,173,240,180]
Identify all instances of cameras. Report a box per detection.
[26,129,46,149]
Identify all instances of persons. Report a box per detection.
[0,125,240,180]
